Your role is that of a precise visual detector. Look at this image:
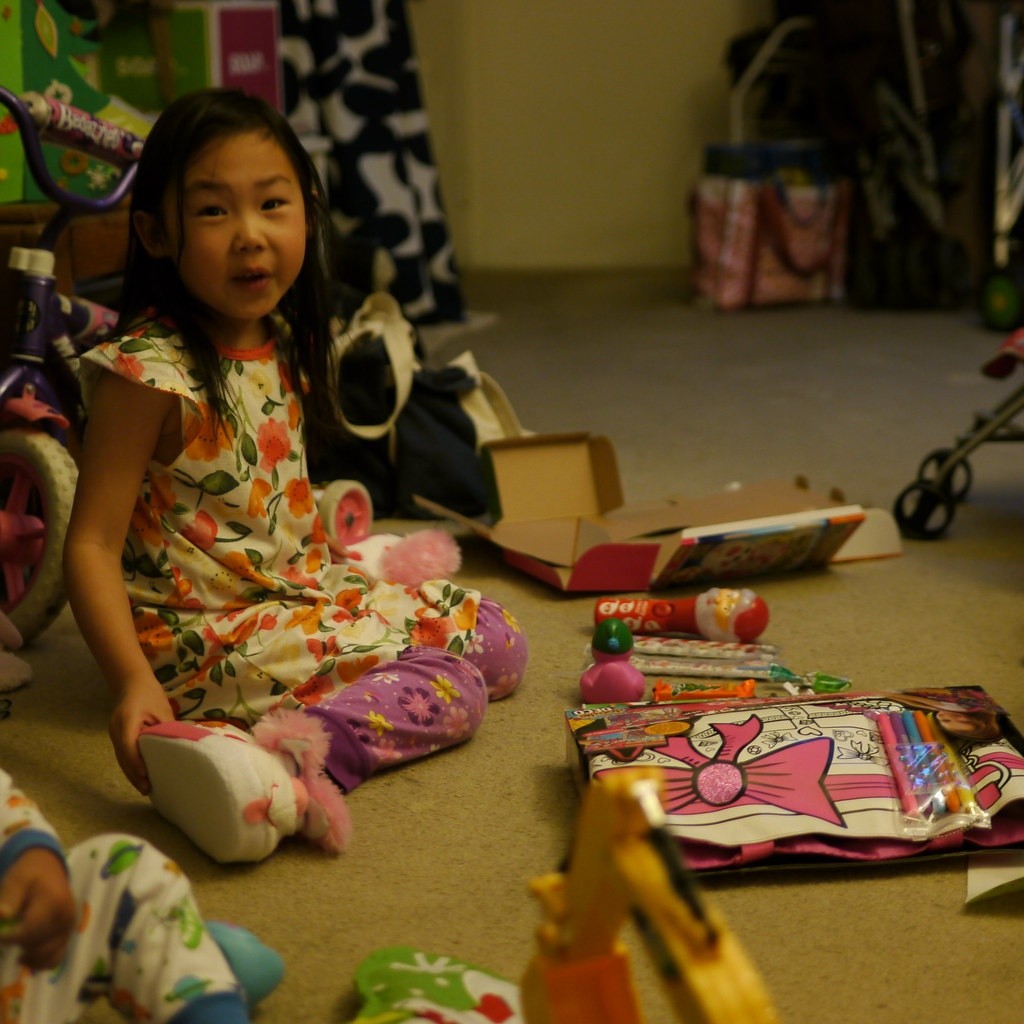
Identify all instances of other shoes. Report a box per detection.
[341,529,463,589]
[138,708,353,863]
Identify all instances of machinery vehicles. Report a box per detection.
[515,764,775,1022]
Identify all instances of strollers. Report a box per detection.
[892,332,1024,541]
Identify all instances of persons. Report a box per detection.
[1,758,288,1023]
[60,84,528,867]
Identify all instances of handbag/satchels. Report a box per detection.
[324,290,537,520]
[688,166,853,304]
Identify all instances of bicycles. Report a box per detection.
[0,85,327,646]
